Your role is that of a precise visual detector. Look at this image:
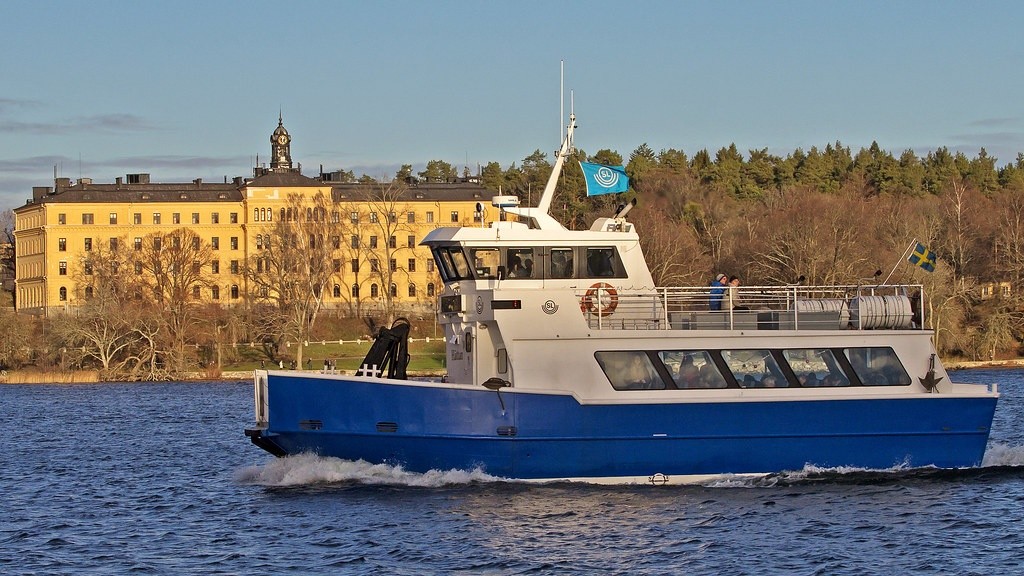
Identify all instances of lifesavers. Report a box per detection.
[584,282,619,317]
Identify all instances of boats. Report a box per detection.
[243,59,1003,483]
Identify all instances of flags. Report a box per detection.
[907,242,937,273]
[578,161,630,196]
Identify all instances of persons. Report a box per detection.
[279,360,283,369]
[552,250,614,277]
[324,359,337,370]
[666,355,723,388]
[261,360,265,370]
[624,355,652,389]
[292,360,296,369]
[710,274,749,313]
[736,374,778,387]
[799,372,843,386]
[861,366,889,385]
[512,257,532,278]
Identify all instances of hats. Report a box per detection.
[729,275,738,282]
[715,274,726,282]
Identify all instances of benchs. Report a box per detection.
[668,312,839,330]
